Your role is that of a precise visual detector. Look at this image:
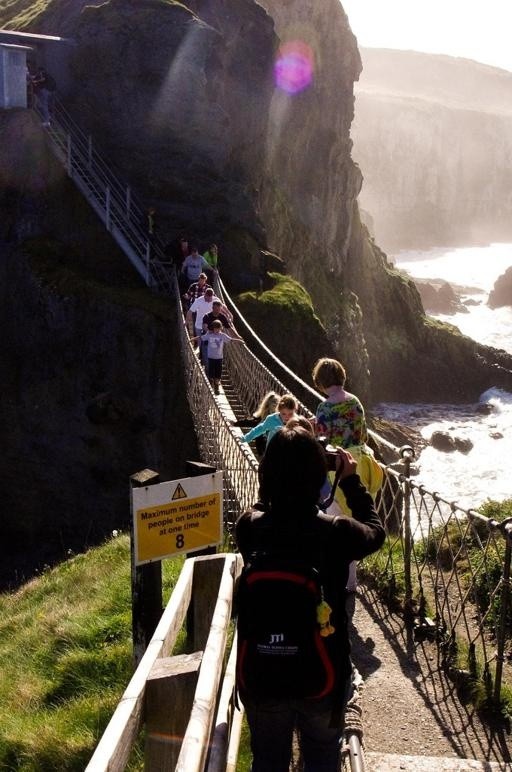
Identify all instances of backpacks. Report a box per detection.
[231,546,352,711]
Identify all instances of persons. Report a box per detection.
[144,207,158,238]
[313,356,370,594]
[223,392,281,455]
[27,59,58,129]
[234,398,299,452]
[231,424,387,772]
[165,234,245,395]
[283,419,341,520]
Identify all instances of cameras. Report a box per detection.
[325,452,341,471]
